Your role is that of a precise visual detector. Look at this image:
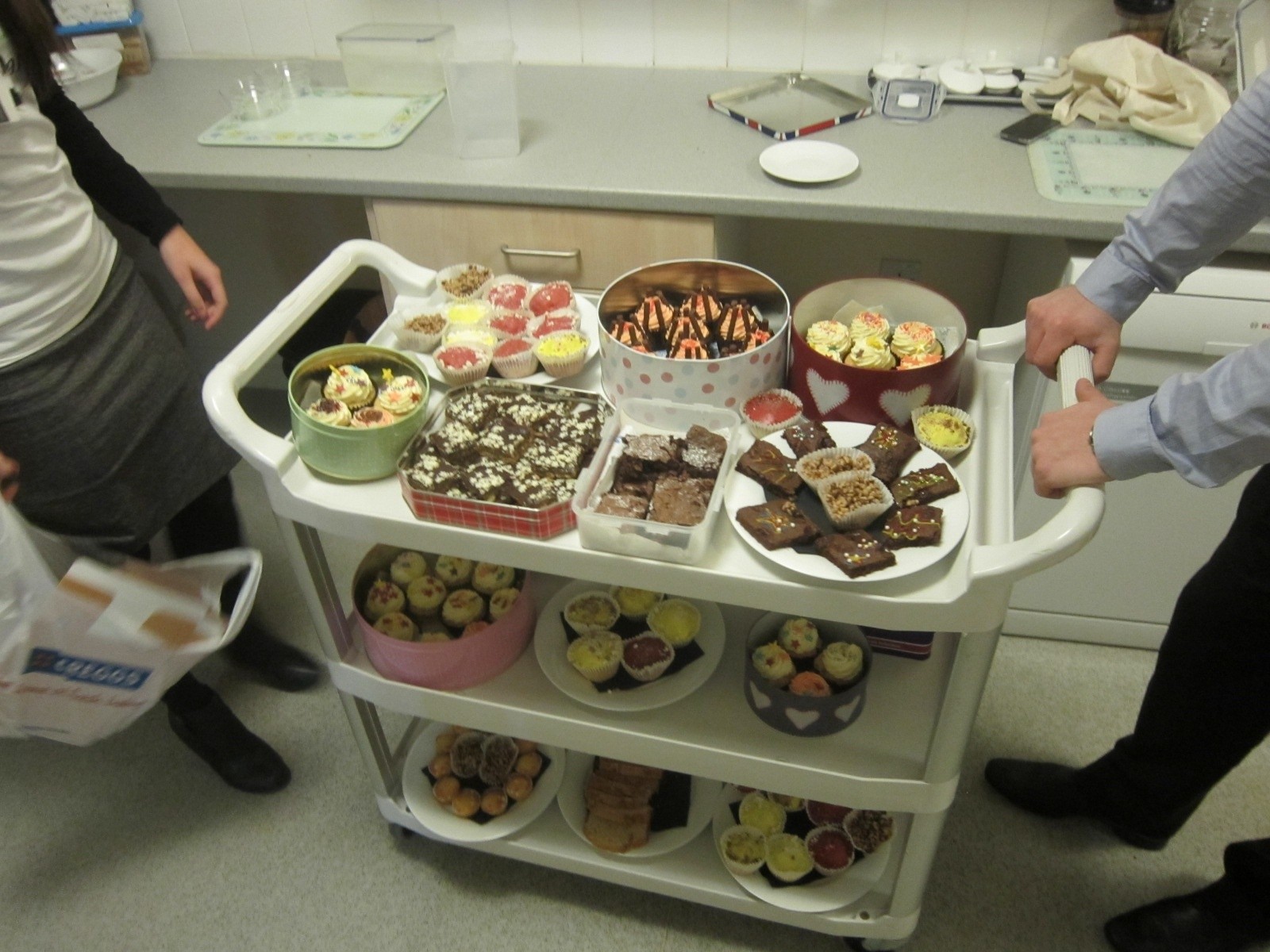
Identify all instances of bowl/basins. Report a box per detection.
[49,48,123,110]
[220,57,314,121]
[287,342,430,481]
[596,257,791,433]
[787,276,969,435]
[742,613,875,740]
[349,543,534,691]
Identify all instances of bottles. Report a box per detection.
[1105,1,1241,78]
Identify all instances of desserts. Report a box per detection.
[805,311,946,371]
[738,388,803,438]
[814,641,863,684]
[611,284,775,360]
[307,364,422,429]
[789,671,832,697]
[911,404,973,461]
[778,617,823,658]
[752,640,796,688]
[394,262,591,388]
[429,724,543,817]
[720,784,897,883]
[364,551,520,642]
[565,584,700,680]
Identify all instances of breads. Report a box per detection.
[583,757,663,852]
[735,421,960,579]
[593,423,727,549]
[406,388,603,508]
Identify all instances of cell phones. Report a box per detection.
[1000,114,1061,145]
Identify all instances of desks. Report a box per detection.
[80,60,1270,254]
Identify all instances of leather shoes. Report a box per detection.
[1106,885,1265,952]
[225,624,324,694]
[168,687,293,795]
[987,759,1133,841]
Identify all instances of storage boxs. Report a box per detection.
[338,23,453,96]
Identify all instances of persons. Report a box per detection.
[0,0,321,793]
[982,67,1270,952]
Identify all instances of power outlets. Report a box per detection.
[880,259,921,282]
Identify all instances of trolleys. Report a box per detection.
[200,238,1106,952]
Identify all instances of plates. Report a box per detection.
[758,140,861,183]
[534,578,728,712]
[401,718,895,914]
[391,287,972,581]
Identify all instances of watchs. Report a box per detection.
[1087,422,1097,462]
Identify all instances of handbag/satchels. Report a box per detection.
[0,497,262,743]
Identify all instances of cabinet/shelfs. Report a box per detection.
[202,239,1105,940]
[362,197,740,317]
